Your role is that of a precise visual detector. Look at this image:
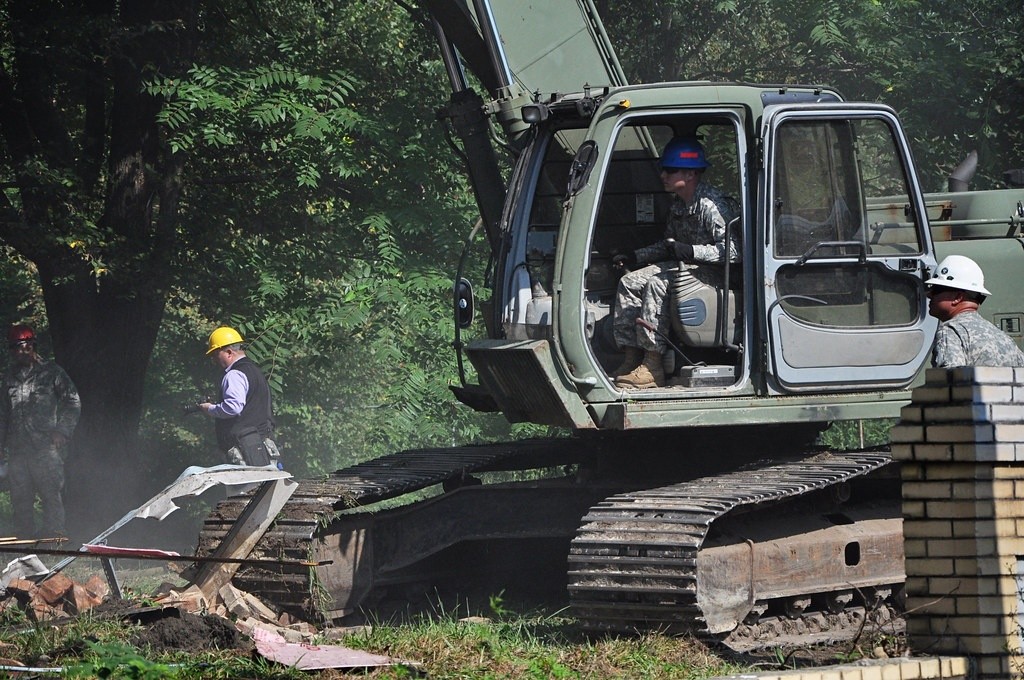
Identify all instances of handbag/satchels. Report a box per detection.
[235,427,270,467]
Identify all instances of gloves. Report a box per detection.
[663,238,693,260]
[609,248,634,275]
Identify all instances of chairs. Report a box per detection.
[606,260,742,351]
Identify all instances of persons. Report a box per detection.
[196,327,280,468]
[613,136,743,389]
[0,324,82,539]
[924,255,1024,368]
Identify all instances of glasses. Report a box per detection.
[659,168,678,174]
[930,285,957,295]
[9,341,26,349]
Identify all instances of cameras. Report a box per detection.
[179,394,217,416]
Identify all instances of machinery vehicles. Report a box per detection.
[188,1,1023,635]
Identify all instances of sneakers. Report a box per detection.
[608,368,633,384]
[616,365,665,389]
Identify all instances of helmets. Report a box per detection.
[924,255,993,296]
[657,135,711,170]
[205,326,243,354]
[8,325,33,340]
